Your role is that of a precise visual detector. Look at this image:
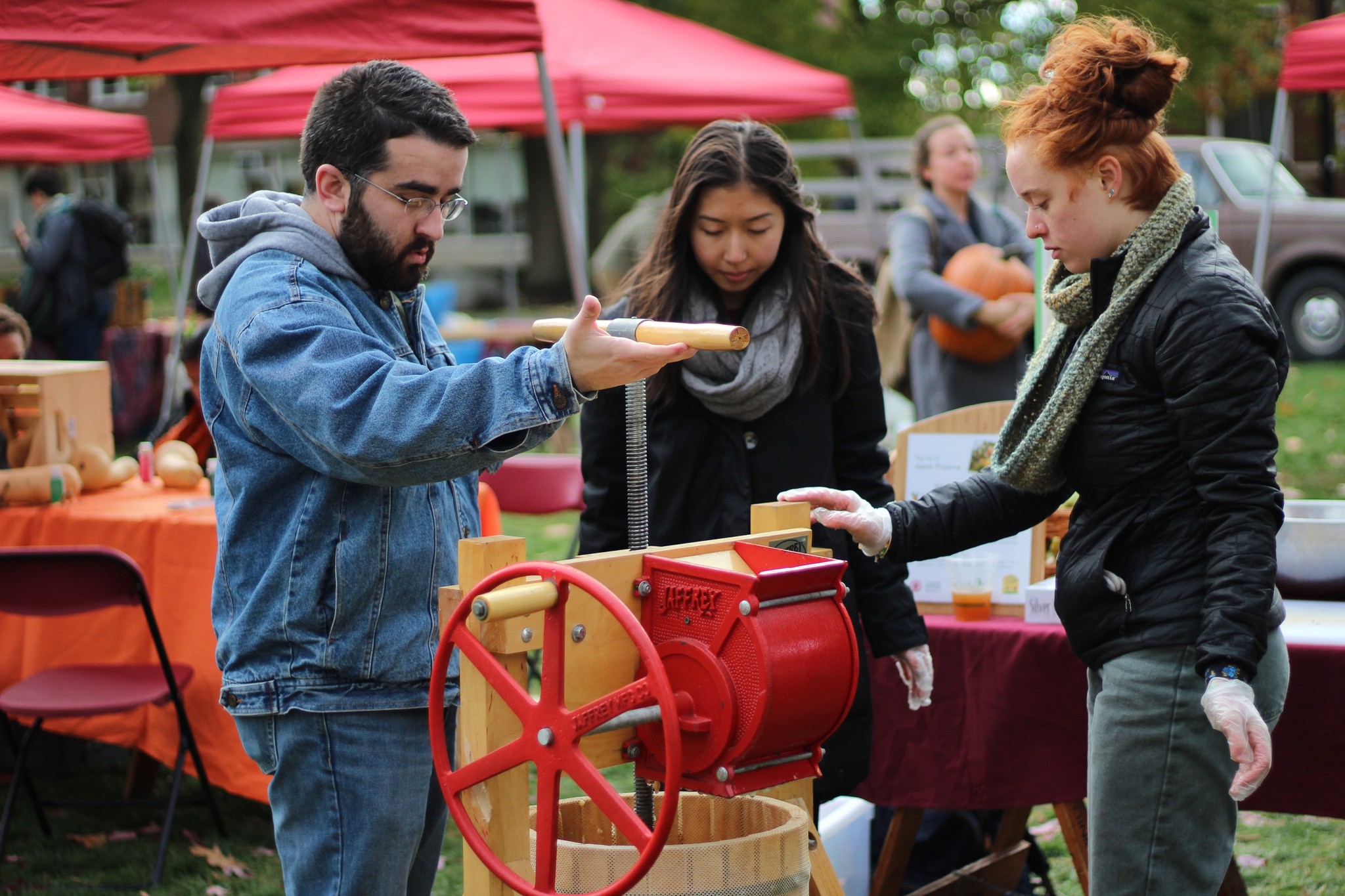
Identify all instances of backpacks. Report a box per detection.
[36,201,131,289]
[869,201,941,399]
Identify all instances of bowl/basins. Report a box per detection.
[1272,499,1344,583]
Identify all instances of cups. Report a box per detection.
[944,556,995,621]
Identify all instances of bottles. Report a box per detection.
[48,466,65,507]
[137,442,156,483]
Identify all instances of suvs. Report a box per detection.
[1166,134,1345,364]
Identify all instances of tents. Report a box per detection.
[1,1,590,311]
[1253,12,1345,290]
[156,0,883,436]
[0,84,179,298]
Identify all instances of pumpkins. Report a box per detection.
[0,442,202,503]
[929,242,1035,361]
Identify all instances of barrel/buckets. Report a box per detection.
[521,790,810,896]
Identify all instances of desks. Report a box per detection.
[860,596,1095,896]
[0,465,587,810]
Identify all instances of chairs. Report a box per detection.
[0,540,231,885]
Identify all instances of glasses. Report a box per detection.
[336,165,468,221]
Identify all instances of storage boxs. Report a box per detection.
[0,357,119,466]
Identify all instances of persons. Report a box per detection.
[888,113,1035,420]
[195,55,697,896]
[580,120,935,839]
[0,303,33,467]
[153,330,220,465]
[777,17,1290,896]
[15,166,128,359]
